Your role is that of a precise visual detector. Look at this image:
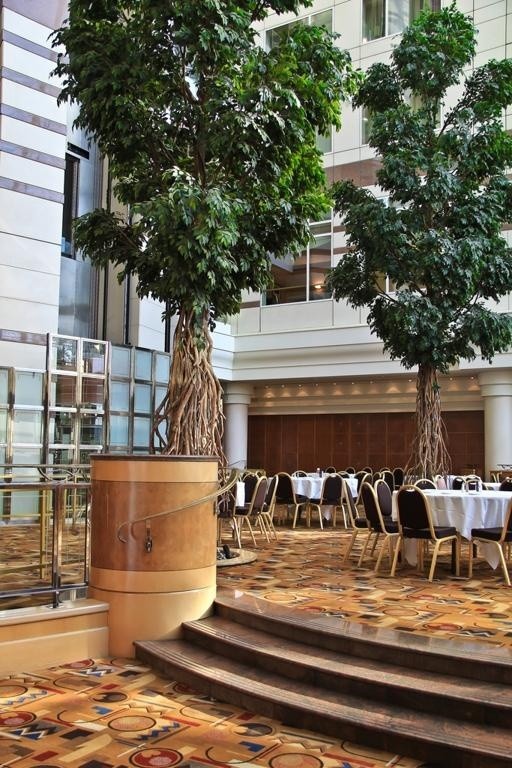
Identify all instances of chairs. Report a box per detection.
[217,465,510,587]
